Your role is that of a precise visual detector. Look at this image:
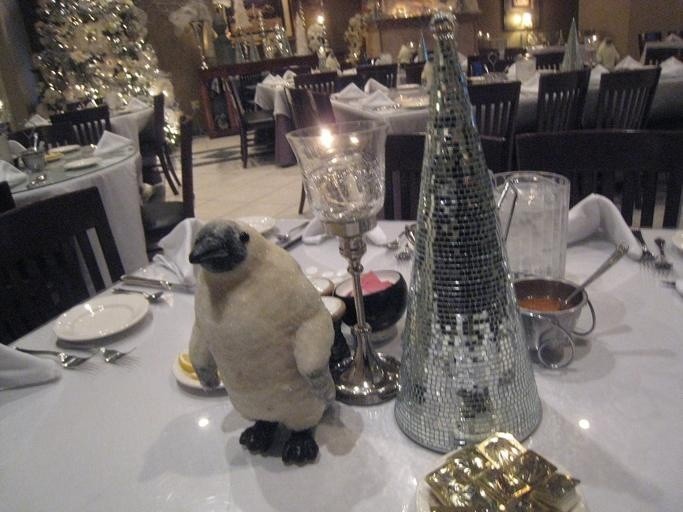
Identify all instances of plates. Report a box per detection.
[11,144,102,184]
[172,350,223,392]
[672,230,682,297]
[240,215,277,234]
[396,81,430,112]
[405,224,417,251]
[54,293,150,342]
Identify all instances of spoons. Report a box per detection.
[386,231,404,249]
[113,288,164,301]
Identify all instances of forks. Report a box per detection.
[654,239,676,287]
[14,345,94,370]
[54,335,136,365]
[629,226,658,274]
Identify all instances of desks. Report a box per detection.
[375,12,482,57]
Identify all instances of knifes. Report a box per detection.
[118,274,190,293]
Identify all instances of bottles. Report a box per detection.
[542,28,597,48]
[393,5,453,17]
[239,23,290,60]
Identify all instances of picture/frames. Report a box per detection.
[512,0,531,8]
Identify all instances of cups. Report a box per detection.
[513,278,589,364]
[489,170,570,279]
[515,57,536,81]
[334,269,409,345]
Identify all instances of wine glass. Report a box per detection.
[286,118,405,402]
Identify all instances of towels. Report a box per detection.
[567,191,645,261]
[301,213,388,246]
[0,344,60,392]
